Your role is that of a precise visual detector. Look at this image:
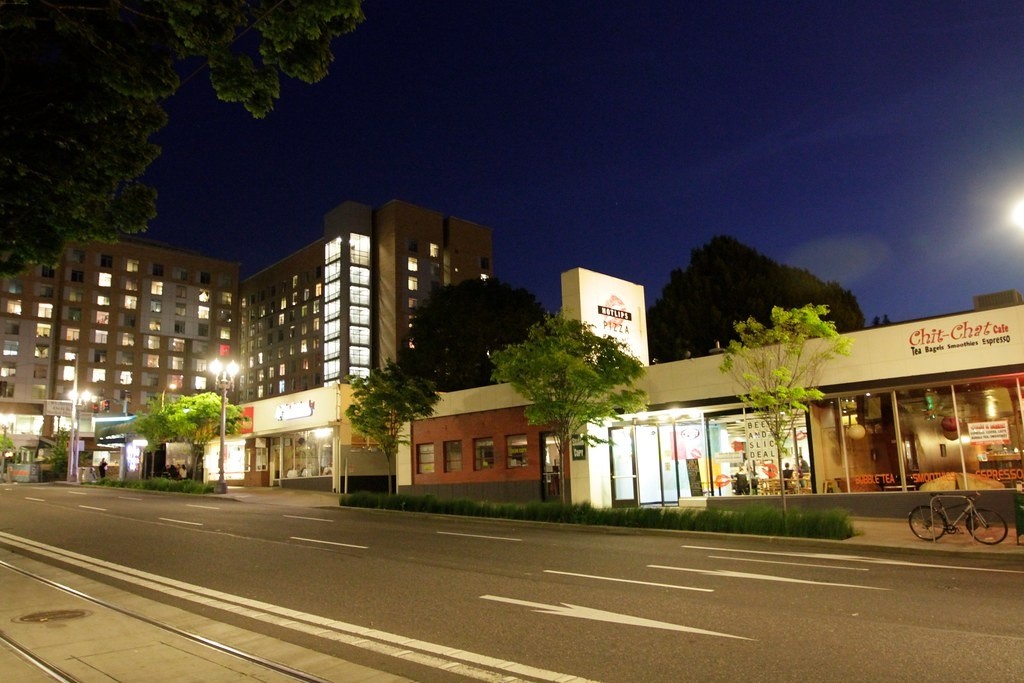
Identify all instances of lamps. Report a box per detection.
[844,424,865,439]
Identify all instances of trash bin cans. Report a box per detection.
[78,467,95,483]
[1015,493,1024,536]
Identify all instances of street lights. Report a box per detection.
[207,357,239,495]
[66,389,91,484]
[0,412,16,484]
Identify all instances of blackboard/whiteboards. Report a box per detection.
[686,459,702,496]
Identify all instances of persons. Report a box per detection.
[296,463,306,476]
[166,464,187,480]
[99,459,107,477]
[785,463,789,470]
[799,455,809,472]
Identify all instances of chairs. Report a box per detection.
[756,473,812,495]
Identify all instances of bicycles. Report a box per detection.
[908,490,1009,547]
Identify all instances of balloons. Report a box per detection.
[942,417,962,440]
[849,424,865,440]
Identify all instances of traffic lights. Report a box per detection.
[93,403,98,413]
[105,399,111,412]
